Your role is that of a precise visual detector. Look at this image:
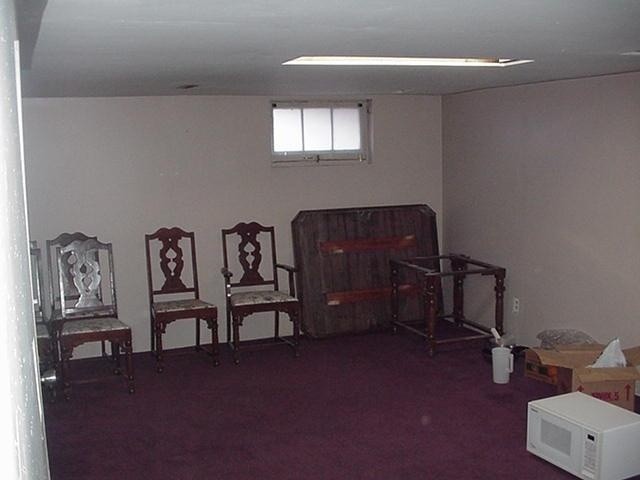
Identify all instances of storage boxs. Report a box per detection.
[522,350,558,384]
[530,347,640,411]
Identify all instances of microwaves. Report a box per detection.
[525,391,640,480]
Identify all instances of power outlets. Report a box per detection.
[512,298,519,313]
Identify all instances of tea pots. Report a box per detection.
[491,347,514,386]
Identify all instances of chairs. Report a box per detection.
[29,233,135,405]
[144,226,219,372]
[222,222,303,365]
